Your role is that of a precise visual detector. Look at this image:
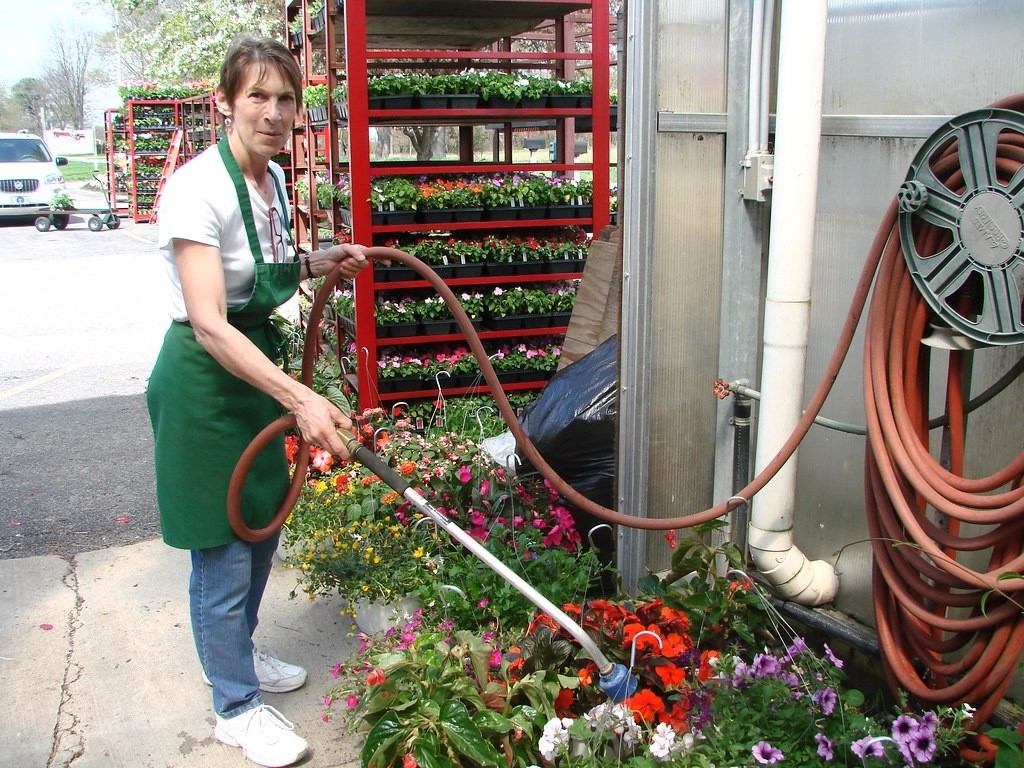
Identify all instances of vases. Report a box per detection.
[302,93,594,121]
[337,312,572,339]
[302,312,357,379]
[378,370,557,396]
[373,256,587,282]
[322,204,593,227]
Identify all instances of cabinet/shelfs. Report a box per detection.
[104,108,128,216]
[281,0,611,410]
[180,87,294,228]
[123,99,220,225]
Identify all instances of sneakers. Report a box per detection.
[214,703,308,768]
[202,638,308,693]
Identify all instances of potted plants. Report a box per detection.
[109,82,295,224]
[287,0,342,49]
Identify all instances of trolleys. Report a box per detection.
[32,174,120,231]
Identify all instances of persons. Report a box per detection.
[139,30,369,767]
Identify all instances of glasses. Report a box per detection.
[268,207,285,264]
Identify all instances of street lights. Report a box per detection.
[112,23,122,108]
[91,114,99,172]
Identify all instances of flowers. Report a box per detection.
[301,69,590,106]
[298,294,357,373]
[333,229,598,269]
[377,336,567,379]
[331,280,581,323]
[298,171,592,214]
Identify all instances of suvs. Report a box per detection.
[0,129,68,225]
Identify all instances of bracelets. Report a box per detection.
[304,250,316,280]
